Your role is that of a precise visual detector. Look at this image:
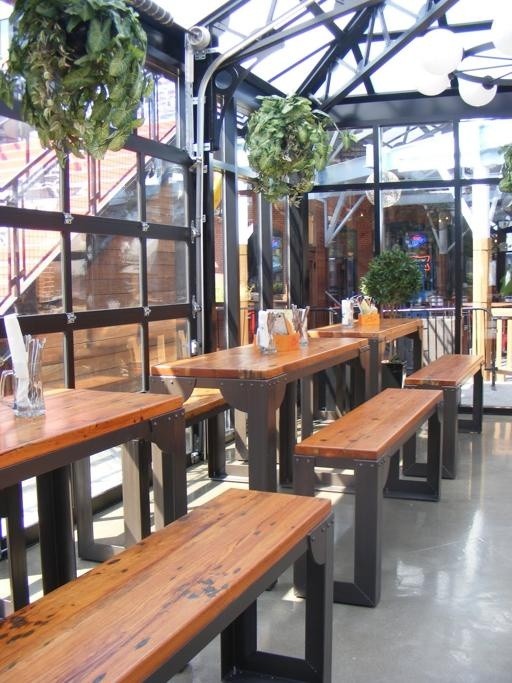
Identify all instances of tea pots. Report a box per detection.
[1,335,48,417]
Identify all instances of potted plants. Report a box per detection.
[358,246,423,389]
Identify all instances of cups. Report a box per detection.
[256,318,277,354]
[294,315,308,345]
[341,299,353,325]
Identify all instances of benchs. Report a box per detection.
[0,488,336,683]
[402,354,486,478]
[180,387,231,480]
[292,388,445,608]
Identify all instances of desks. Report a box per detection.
[150,339,371,592]
[0,386,185,622]
[302,313,423,442]
